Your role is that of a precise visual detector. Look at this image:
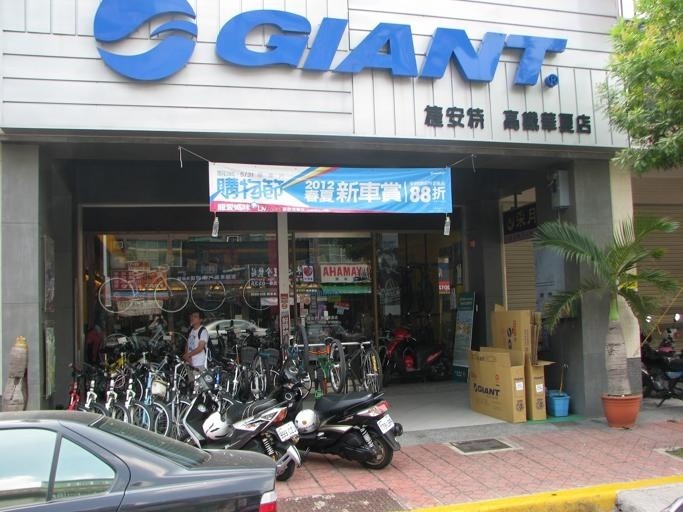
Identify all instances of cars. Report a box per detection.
[0,408,280,512]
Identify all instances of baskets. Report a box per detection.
[241,346,257,364]
[265,349,280,366]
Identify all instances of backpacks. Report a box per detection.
[188,326,215,362]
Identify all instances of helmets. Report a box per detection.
[203,412,234,442]
[295,409,320,434]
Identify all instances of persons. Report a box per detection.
[148,315,167,336]
[180,310,209,374]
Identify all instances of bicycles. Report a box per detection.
[64,320,384,445]
[191,264,280,312]
[96,263,191,313]
[395,265,438,348]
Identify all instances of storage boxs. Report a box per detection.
[467,304,556,421]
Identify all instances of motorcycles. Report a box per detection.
[181,362,304,483]
[640,313,683,408]
[248,356,404,470]
[384,323,452,380]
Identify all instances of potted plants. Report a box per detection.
[530,214,683,430]
